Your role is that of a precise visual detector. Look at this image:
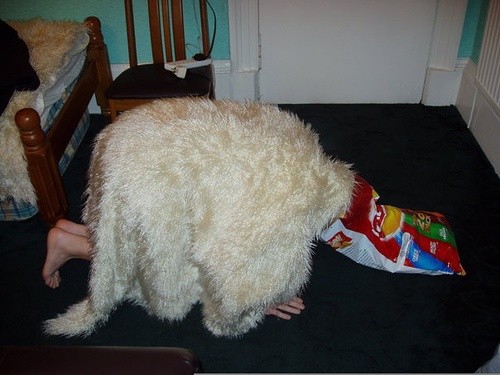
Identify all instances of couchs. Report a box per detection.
[0,345,199,375]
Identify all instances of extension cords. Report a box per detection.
[164,58,212,72]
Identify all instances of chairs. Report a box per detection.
[105,1,212,119]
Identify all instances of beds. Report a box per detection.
[0,17,116,226]
